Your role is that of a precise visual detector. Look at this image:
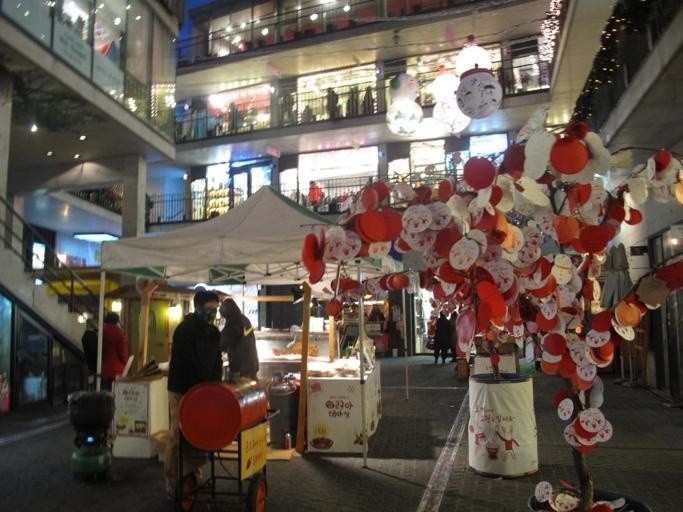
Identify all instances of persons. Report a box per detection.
[301,106,314,125]
[350,304,358,319]
[363,85,375,116]
[368,304,386,333]
[325,87,338,121]
[346,87,359,118]
[218,297,260,388]
[433,311,452,366]
[447,311,460,362]
[158,290,226,511]
[307,180,320,214]
[97,311,130,392]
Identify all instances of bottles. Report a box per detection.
[284,431,291,450]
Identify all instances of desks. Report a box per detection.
[112,355,381,459]
